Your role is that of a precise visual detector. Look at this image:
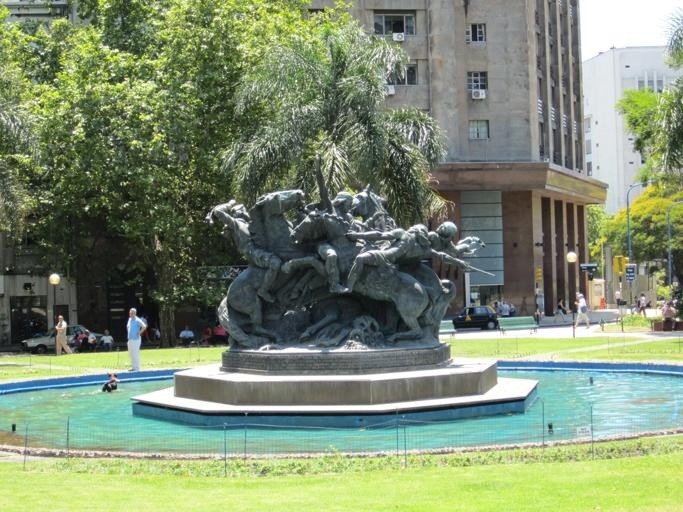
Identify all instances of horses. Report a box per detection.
[348,183,457,339]
[280,207,436,347]
[217,189,320,347]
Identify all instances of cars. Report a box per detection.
[443,306,498,330]
[19,325,104,354]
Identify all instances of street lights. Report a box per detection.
[627,179,656,312]
[49,273,61,355]
[567,251,579,337]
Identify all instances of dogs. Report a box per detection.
[102,373,117,392]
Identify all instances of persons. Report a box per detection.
[572,294,590,330]
[613,289,620,310]
[492,302,500,315]
[213,322,227,346]
[124,307,147,372]
[499,301,510,318]
[210,198,282,304]
[195,325,213,346]
[638,292,647,317]
[278,189,354,295]
[178,324,193,347]
[53,314,72,356]
[661,301,676,332]
[551,296,568,324]
[632,296,640,313]
[509,302,515,316]
[343,218,473,298]
[100,329,115,353]
[73,333,89,353]
[85,329,97,353]
[139,313,153,345]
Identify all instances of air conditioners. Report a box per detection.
[384,85,395,96]
[392,33,405,41]
[472,89,486,99]
